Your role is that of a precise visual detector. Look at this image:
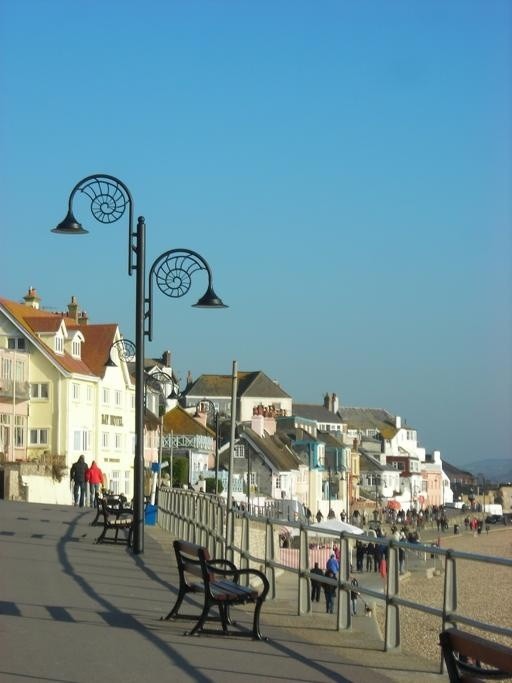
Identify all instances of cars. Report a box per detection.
[485,514,505,524]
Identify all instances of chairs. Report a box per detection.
[438,629,512,683]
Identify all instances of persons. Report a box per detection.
[303,501,493,541]
[351,578,360,615]
[70,454,88,507]
[310,561,324,602]
[322,569,338,614]
[308,542,406,576]
[85,460,105,508]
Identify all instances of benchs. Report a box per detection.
[160,539,271,643]
[92,488,134,547]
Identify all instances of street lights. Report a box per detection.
[311,452,490,521]
[51,174,230,556]
[192,398,242,500]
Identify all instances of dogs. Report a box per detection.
[364,604,375,618]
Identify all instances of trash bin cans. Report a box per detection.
[145,504,157,526]
